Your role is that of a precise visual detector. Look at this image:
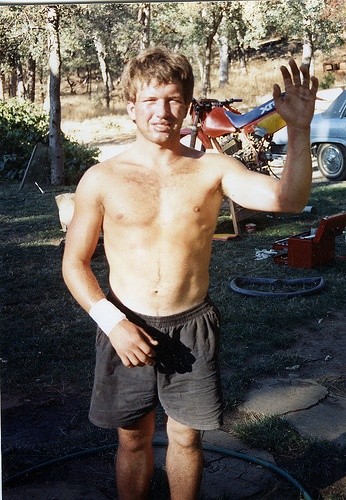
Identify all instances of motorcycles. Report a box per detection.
[179,97,286,180]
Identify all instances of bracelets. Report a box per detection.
[89,299,126,335]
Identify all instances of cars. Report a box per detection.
[310,88,346,181]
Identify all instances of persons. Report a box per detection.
[60,45,318,500]
